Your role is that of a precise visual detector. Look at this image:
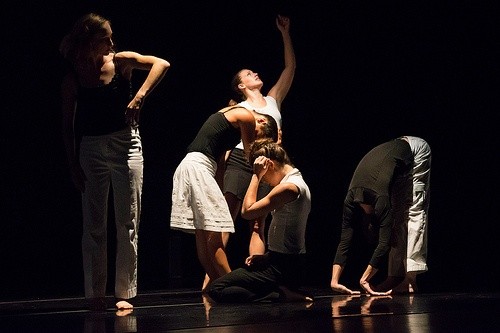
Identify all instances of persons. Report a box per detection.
[205,137,315,304]
[201,14,297,293]
[167,105,279,283]
[61,13,172,314]
[329,135,434,297]
